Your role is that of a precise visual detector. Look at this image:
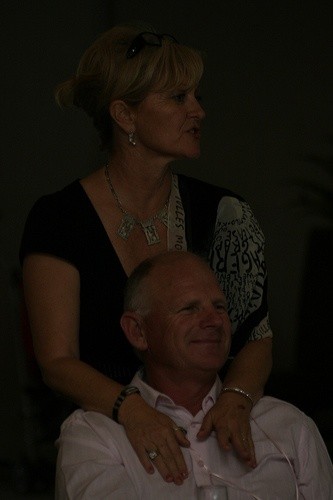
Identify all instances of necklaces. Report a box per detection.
[104,156,174,247]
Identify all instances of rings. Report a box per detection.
[148,449,160,460]
[172,426,187,434]
[242,438,247,441]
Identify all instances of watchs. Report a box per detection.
[112,385,141,423]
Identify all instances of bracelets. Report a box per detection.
[219,386,257,411]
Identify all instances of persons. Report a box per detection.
[51,249,332,500]
[19,25,274,486]
